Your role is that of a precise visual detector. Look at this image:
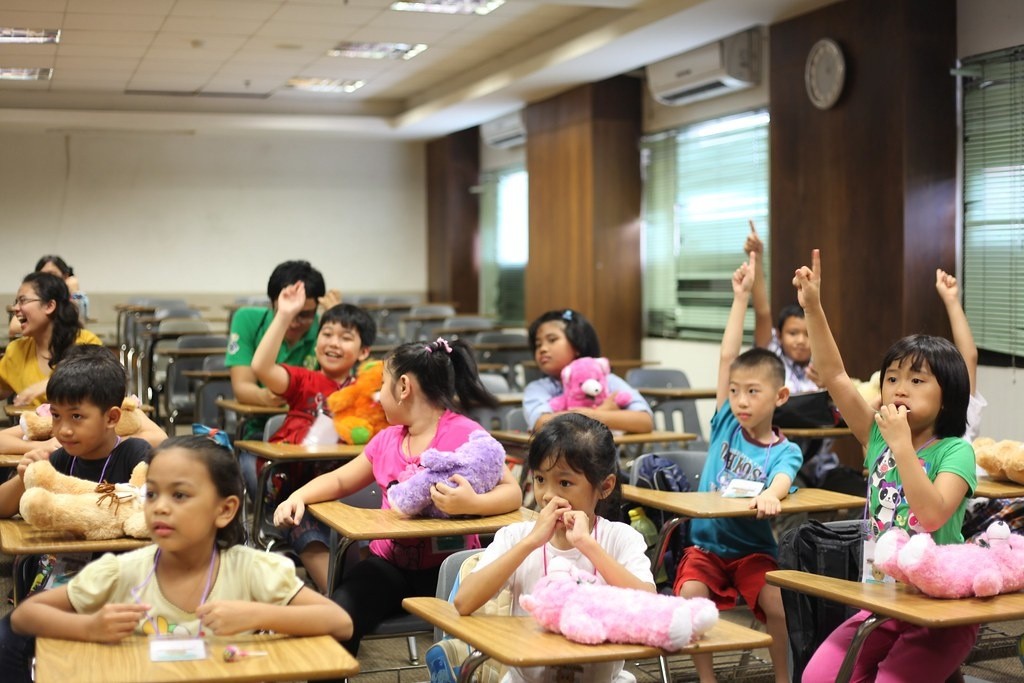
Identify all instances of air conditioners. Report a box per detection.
[644,29,761,108]
[479,109,527,151]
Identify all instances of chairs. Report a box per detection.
[0,294,1024,682]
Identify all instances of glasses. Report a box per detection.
[14,297,39,306]
[298,301,318,324]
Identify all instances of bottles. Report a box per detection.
[628,507,668,584]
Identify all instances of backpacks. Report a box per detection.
[777,518,866,651]
[772,391,847,429]
[962,496,1024,546]
[628,454,695,581]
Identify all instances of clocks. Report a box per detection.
[804,39,848,112]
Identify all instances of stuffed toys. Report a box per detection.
[387,431,506,520]
[972,437,1024,486]
[517,556,720,652]
[17,459,153,542]
[326,358,394,447]
[19,392,142,441]
[873,518,1024,601]
[548,356,631,437]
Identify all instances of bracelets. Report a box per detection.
[70,293,82,300]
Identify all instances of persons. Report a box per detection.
[224,259,343,503]
[249,280,377,593]
[670,250,792,683]
[8,422,355,645]
[791,248,982,683]
[742,218,867,501]
[0,354,156,582]
[521,308,654,436]
[0,272,102,404]
[8,256,88,339]
[934,268,987,451]
[453,413,660,683]
[272,338,523,683]
[0,344,168,454]
